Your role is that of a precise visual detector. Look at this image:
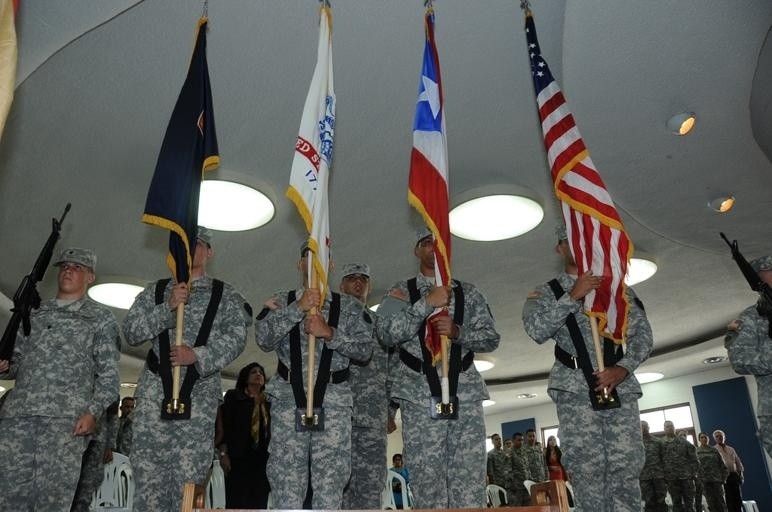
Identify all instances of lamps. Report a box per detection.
[622,251,659,287]
[473,354,497,373]
[196,167,279,233]
[710,196,737,213]
[665,114,695,137]
[86,275,145,309]
[448,184,545,240]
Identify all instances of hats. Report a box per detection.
[53,249,98,272]
[555,223,569,242]
[197,225,211,248]
[300,241,312,256]
[749,255,772,272]
[414,226,434,248]
[341,262,370,281]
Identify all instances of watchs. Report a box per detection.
[220,451,229,456]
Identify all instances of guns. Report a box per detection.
[719,232,772,338]
[1,203,71,373]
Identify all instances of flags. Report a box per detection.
[520,1,636,346]
[286,0,339,320]
[141,0,224,292]
[408,1,453,367]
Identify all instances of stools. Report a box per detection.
[742,501,759,512]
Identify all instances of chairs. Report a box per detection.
[524,480,536,497]
[563,481,575,512]
[206,460,228,510]
[486,484,507,508]
[89,451,133,512]
[380,469,409,512]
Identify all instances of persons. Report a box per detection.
[390,454,409,509]
[521,223,653,512]
[339,264,399,452]
[254,236,374,510]
[118,397,135,457]
[214,362,271,509]
[70,395,121,512]
[376,225,501,510]
[488,429,565,505]
[121,226,252,512]
[0,248,121,512]
[638,420,745,512]
[724,256,772,458]
